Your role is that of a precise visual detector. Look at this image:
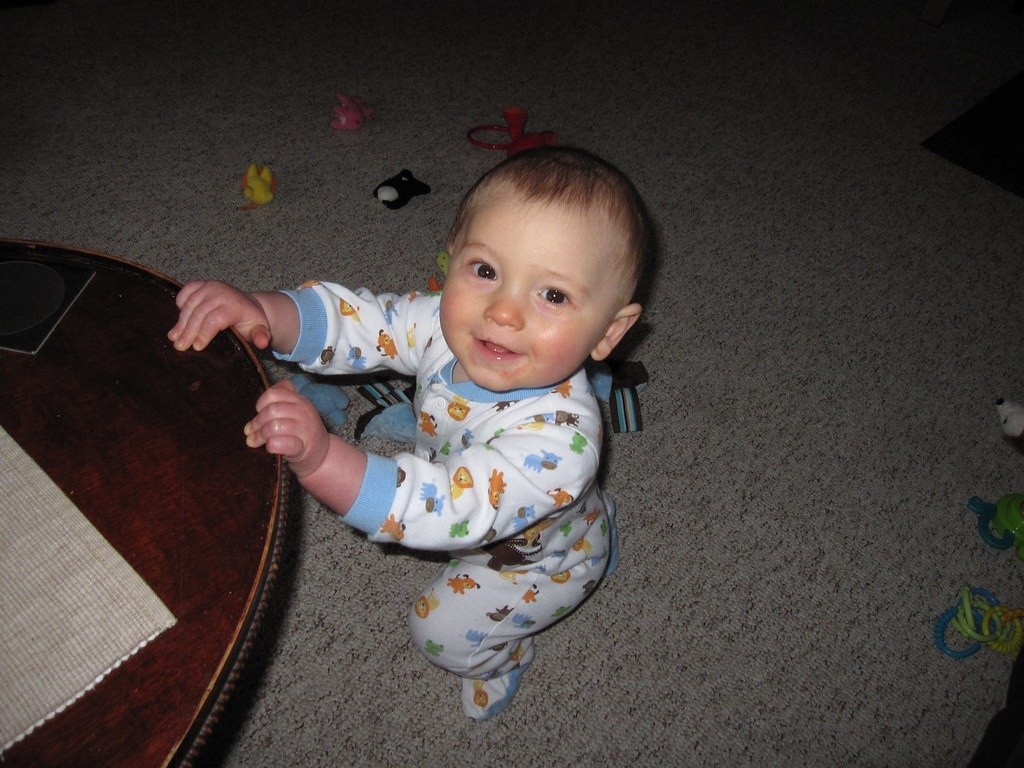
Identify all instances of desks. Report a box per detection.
[0,239,290,768]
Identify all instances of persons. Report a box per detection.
[167,142,651,722]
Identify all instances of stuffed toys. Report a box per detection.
[373,169,431,210]
[239,162,276,204]
[330,91,372,130]
[995,397,1024,437]
